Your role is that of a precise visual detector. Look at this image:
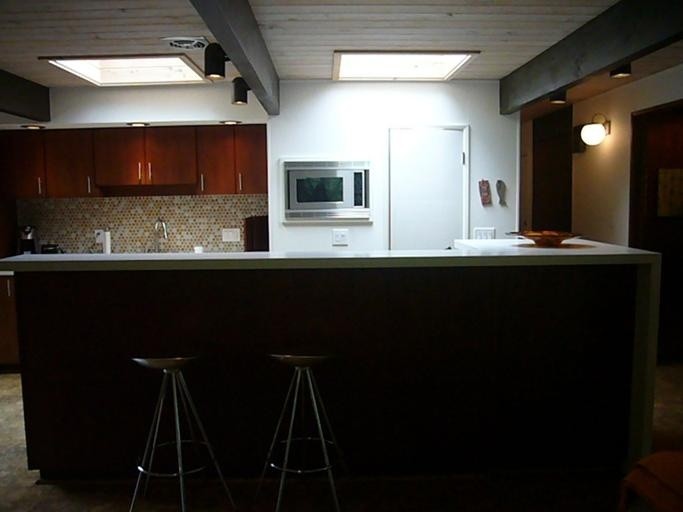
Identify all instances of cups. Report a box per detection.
[194,246,203,253]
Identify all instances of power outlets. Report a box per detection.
[473,227,495,239]
[96,230,103,243]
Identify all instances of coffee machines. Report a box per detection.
[20,226,39,254]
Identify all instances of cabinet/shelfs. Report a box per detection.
[94,125,196,197]
[196,123,267,195]
[0,128,94,199]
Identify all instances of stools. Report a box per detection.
[115,343,240,511]
[252,351,349,512]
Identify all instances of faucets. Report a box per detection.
[154,218,168,253]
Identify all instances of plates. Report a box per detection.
[505,230,583,247]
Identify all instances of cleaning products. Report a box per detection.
[101,225,112,253]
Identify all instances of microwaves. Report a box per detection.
[284,161,370,219]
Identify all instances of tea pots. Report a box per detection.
[40,241,65,253]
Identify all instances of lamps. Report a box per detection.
[581,121,611,146]
[610,64,633,79]
[204,43,230,79]
[231,77,251,105]
[550,93,565,106]
